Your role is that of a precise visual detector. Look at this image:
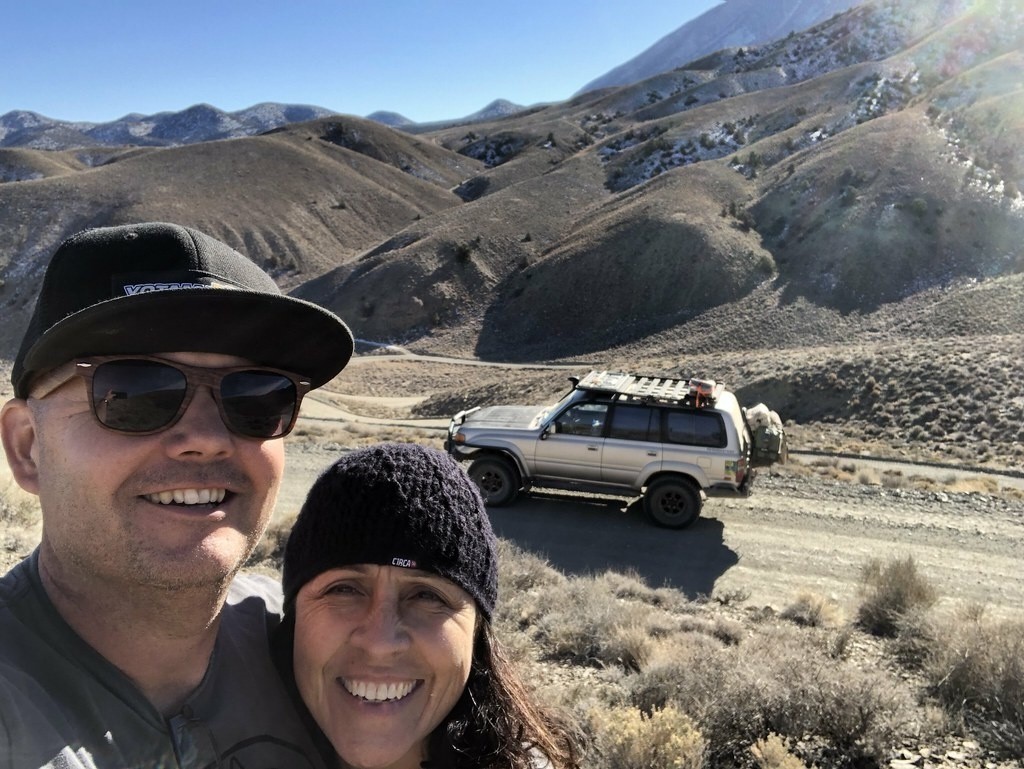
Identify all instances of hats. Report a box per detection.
[282,442,498,614]
[11,223,355,399]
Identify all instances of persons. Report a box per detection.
[0,222,353,769]
[278,442,588,769]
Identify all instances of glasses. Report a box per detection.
[27,355,310,441]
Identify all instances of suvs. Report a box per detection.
[444,370,755,530]
